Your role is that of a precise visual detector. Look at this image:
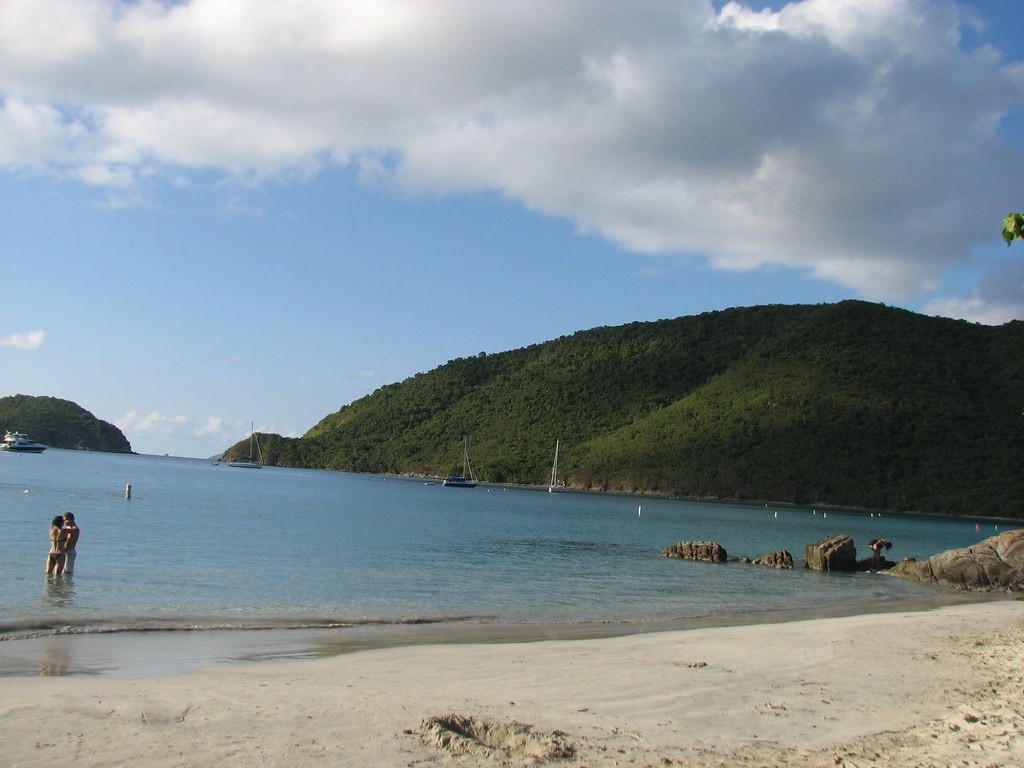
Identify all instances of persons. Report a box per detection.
[46,512,80,578]
[869,538,892,562]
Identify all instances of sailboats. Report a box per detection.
[227,421,264,468]
[441,440,478,488]
[547,438,567,493]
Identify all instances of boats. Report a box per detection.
[0,430,47,453]
